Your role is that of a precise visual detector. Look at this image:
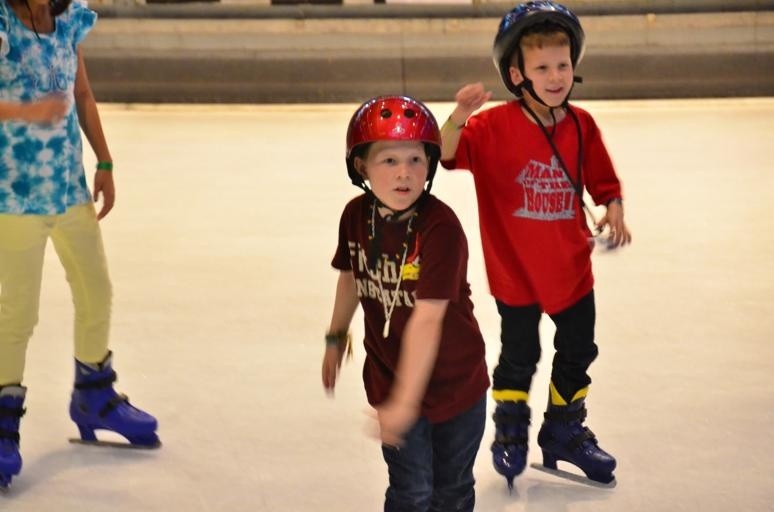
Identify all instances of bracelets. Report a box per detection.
[326,333,353,359]
[448,115,465,130]
[96,161,113,171]
[607,198,622,204]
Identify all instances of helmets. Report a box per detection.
[345,95,442,186]
[492,1,586,96]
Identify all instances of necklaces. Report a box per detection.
[372,197,415,339]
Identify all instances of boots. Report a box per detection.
[490,388,532,477]
[68,352,157,437]
[537,376,617,475]
[1,384,28,477]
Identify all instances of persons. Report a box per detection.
[0,0,160,491]
[322,97,490,511]
[439,0,631,490]
